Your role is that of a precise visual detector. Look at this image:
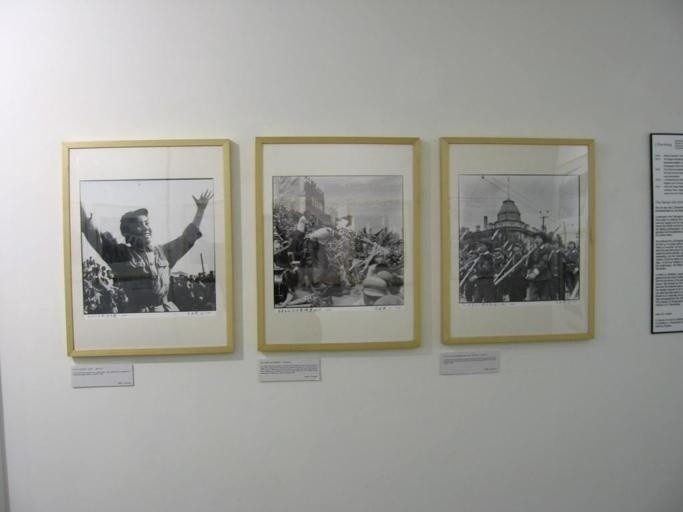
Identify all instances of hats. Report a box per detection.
[120,209,147,232]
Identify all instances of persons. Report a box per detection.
[273,205,403,309]
[80,188,213,314]
[460,230,578,303]
